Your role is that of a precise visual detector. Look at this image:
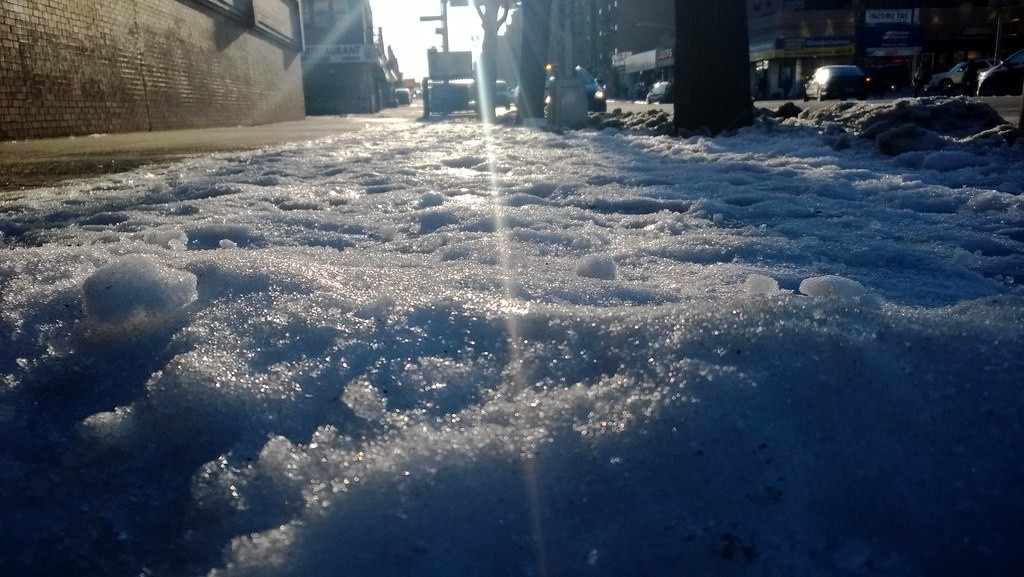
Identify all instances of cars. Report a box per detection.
[646,82,672,104]
[494,80,512,111]
[976,48,1024,97]
[802,64,872,102]
[931,60,1002,95]
[514,67,608,112]
